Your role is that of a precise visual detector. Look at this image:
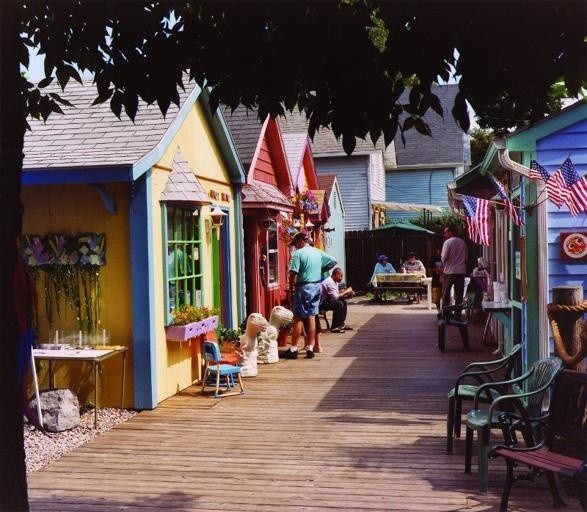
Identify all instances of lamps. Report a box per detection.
[204,203,227,241]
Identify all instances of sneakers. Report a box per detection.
[330,328,345,333]
[280,348,298,360]
[340,326,353,330]
[307,349,315,358]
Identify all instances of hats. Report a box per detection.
[290,233,308,245]
[379,255,388,261]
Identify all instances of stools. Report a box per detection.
[318,310,330,331]
[207,357,239,388]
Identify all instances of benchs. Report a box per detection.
[374,286,424,304]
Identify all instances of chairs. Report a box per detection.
[464,357,564,493]
[487,369,587,512]
[437,297,471,350]
[200,341,244,398]
[447,343,524,454]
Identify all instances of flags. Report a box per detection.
[463,195,491,248]
[530,160,549,183]
[546,158,581,209]
[562,175,586,217]
[487,175,525,228]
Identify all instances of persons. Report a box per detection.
[319,268,355,334]
[279,232,338,359]
[439,224,469,321]
[399,251,426,304]
[429,241,445,320]
[367,254,397,302]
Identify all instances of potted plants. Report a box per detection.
[294,190,318,214]
[166,304,220,342]
[238,305,292,364]
[217,312,267,378]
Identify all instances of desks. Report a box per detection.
[30,344,128,429]
[376,281,421,301]
